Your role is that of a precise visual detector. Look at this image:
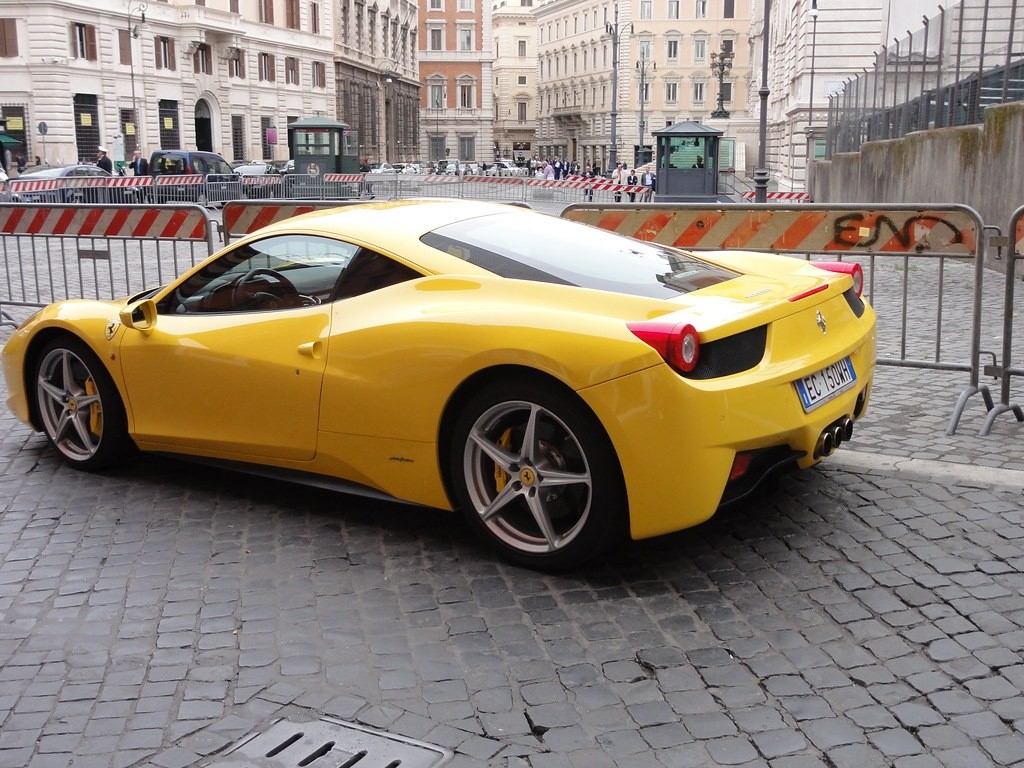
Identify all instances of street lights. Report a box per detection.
[604,21,634,179]
[127,9,148,150]
[377,61,394,167]
[806,9,820,164]
[710,44,734,119]
[635,60,657,149]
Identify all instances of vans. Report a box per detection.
[148,150,243,203]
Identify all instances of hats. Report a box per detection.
[95,145,108,153]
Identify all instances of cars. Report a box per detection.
[230,164,294,200]
[445,147,450,156]
[487,160,522,175]
[229,158,266,166]
[279,160,294,174]
[460,161,482,175]
[435,160,460,176]
[367,159,434,174]
[12,165,139,203]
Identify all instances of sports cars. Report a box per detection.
[0,198,876,573]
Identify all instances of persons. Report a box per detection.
[627,170,638,202]
[561,159,569,179]
[581,166,595,202]
[517,152,525,161]
[358,158,376,199]
[96,146,112,174]
[692,156,704,168]
[5,148,42,172]
[442,160,486,175]
[527,155,580,181]
[612,163,627,202]
[641,166,656,202]
[586,159,590,166]
[129,151,147,203]
[406,161,415,168]
